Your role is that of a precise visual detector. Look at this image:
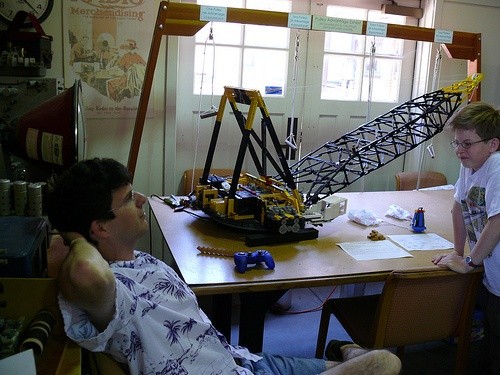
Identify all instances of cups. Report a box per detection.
[28,183,42,216]
[12,181,27,217]
[0,179,11,217]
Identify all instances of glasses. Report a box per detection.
[448,137,489,149]
[101,188,135,218]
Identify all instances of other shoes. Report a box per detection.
[325,338,356,362]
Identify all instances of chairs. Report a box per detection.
[183,169,234,196]
[314,268,474,375]
[395,171,446,191]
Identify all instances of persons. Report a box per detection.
[432,102,500,375]
[48,156,402,375]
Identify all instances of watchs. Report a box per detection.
[465,256,480,267]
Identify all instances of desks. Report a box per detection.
[148,189,483,353]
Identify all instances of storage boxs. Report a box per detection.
[0,215,51,279]
